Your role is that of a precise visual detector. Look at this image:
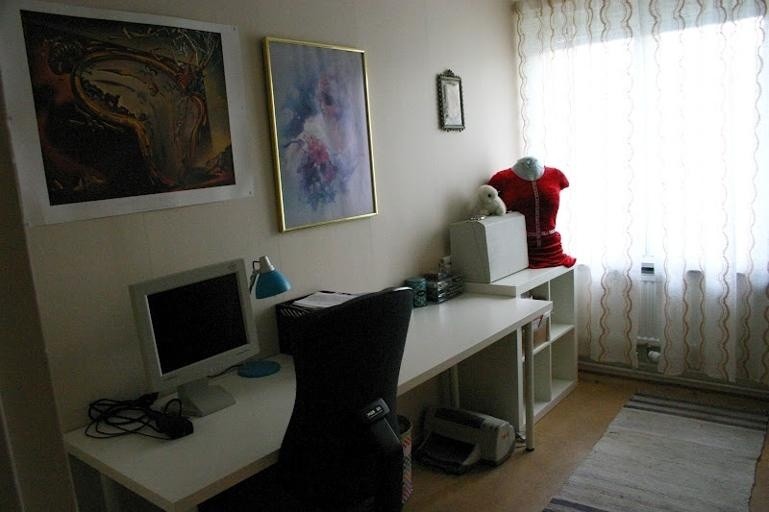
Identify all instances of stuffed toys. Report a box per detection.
[468,185,507,217]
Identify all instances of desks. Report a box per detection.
[63,294,553,512]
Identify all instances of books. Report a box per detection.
[292,291,355,309]
[421,272,466,303]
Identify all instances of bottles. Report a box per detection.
[405,276,428,308]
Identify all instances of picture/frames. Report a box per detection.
[437,69,465,133]
[0,0,257,227]
[262,35,380,233]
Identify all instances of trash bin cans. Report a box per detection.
[398,414,413,504]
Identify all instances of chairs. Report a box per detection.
[275,286,415,512]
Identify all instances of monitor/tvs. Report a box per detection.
[127,257,261,415]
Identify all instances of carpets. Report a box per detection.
[538,392,769,511]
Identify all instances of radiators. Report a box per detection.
[635,274,660,347]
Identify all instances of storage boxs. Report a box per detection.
[448,212,530,283]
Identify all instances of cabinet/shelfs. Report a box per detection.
[458,263,579,434]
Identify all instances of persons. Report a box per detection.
[487,156,577,268]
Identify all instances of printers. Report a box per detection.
[413,404,516,475]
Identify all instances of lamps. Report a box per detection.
[239,255,292,378]
